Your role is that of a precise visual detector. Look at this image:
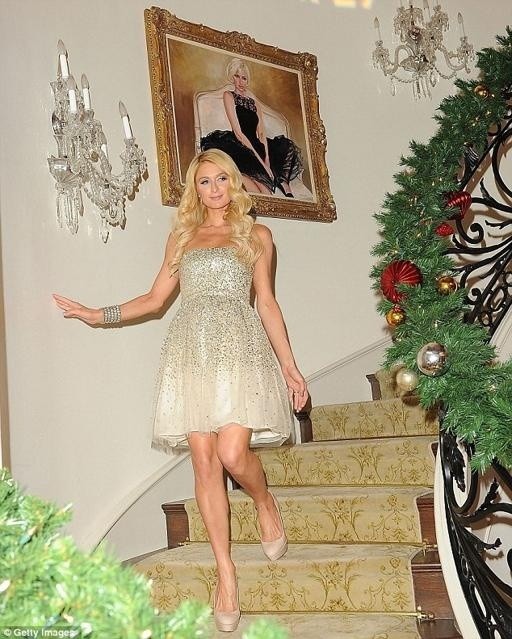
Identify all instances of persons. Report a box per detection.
[51,144,311,634]
[199,57,305,199]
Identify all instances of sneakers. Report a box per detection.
[260,490,289,562]
[213,577,242,633]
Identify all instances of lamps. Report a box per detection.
[372,1,476,102]
[45,37,148,244]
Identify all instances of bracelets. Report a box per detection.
[102,303,122,324]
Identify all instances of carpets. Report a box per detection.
[117,357,441,639]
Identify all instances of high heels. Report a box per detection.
[273,175,295,198]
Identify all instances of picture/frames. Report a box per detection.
[141,7,338,225]
